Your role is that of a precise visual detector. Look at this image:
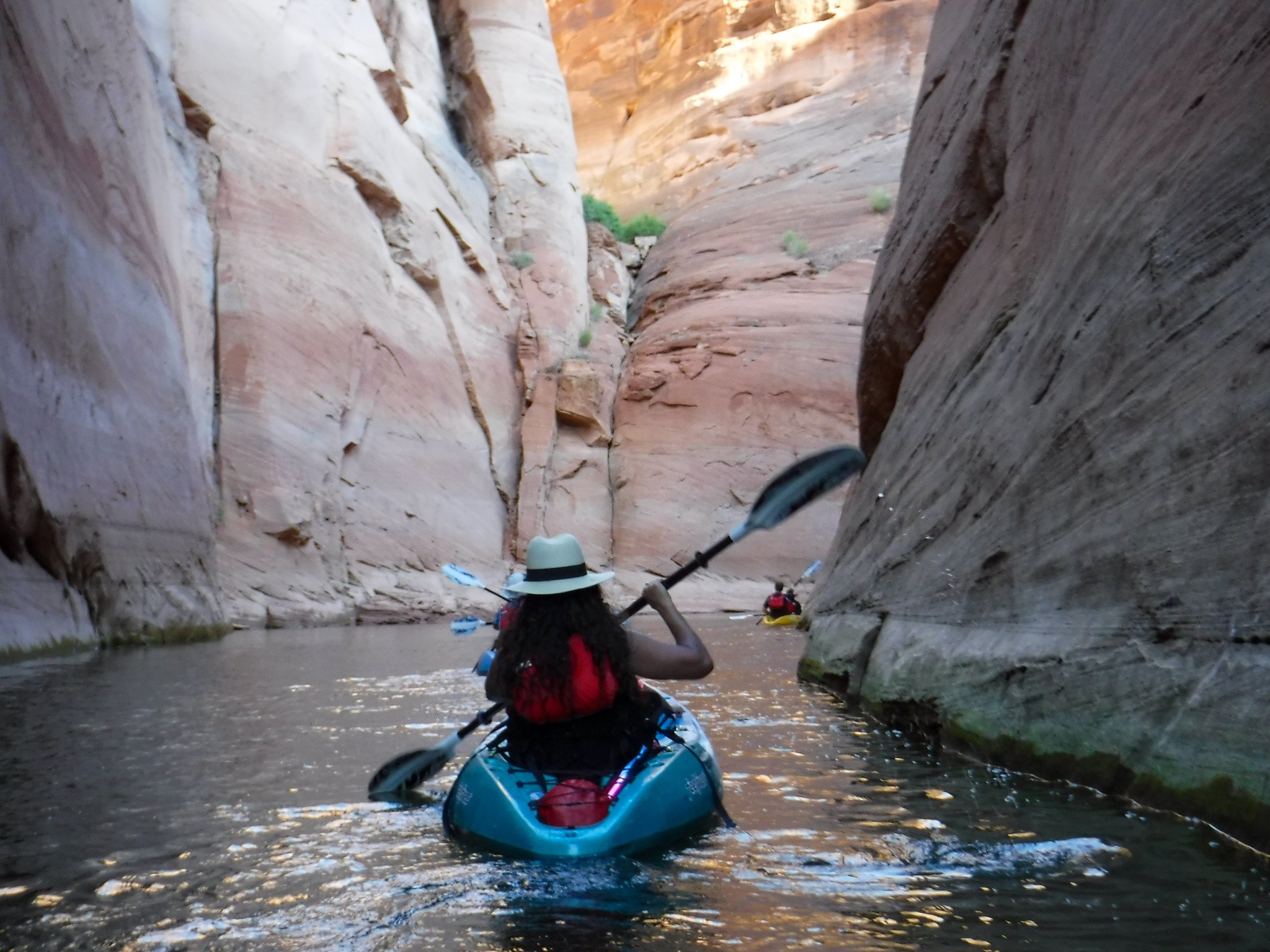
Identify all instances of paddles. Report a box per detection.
[442,563,512,607]
[367,448,880,795]
[754,560,821,626]
[728,613,771,622]
[448,613,497,638]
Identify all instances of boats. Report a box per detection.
[441,675,724,865]
[761,609,802,628]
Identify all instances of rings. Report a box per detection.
[645,581,650,586]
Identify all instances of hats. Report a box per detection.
[501,533,615,595]
[503,572,526,600]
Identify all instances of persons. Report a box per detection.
[484,533,715,782]
[762,581,802,619]
